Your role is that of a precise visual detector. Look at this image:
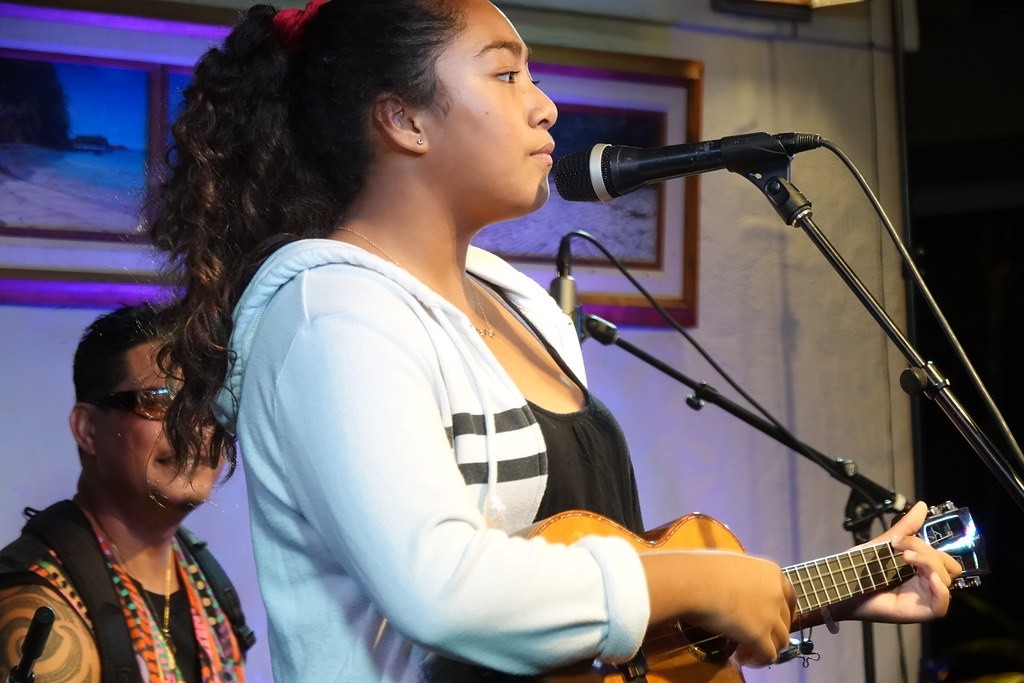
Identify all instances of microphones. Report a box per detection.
[550,237,577,326]
[554,131,823,203]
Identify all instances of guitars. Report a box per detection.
[438,499,989,683]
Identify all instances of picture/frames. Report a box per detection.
[0,1,711,333]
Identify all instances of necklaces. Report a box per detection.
[333,223,497,338]
[103,533,176,655]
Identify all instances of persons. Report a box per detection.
[144,0,961,682]
[0,303,249,683]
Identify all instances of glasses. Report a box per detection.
[74,388,185,421]
